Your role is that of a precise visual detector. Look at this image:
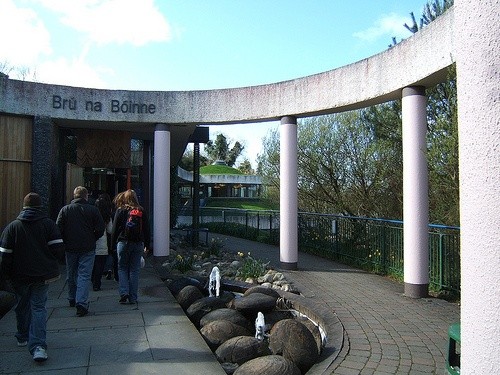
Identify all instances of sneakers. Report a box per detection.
[32,346,49,361]
[16,337,27,346]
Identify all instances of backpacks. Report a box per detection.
[125,206,144,242]
[14,218,61,284]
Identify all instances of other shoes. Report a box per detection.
[76,305,88,317]
[69,299,76,307]
[119,294,128,304]
[105,270,112,280]
[93,287,100,291]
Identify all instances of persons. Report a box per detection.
[0,192,64,360]
[90,193,113,291]
[57,185,104,315]
[108,191,131,281]
[110,189,151,303]
